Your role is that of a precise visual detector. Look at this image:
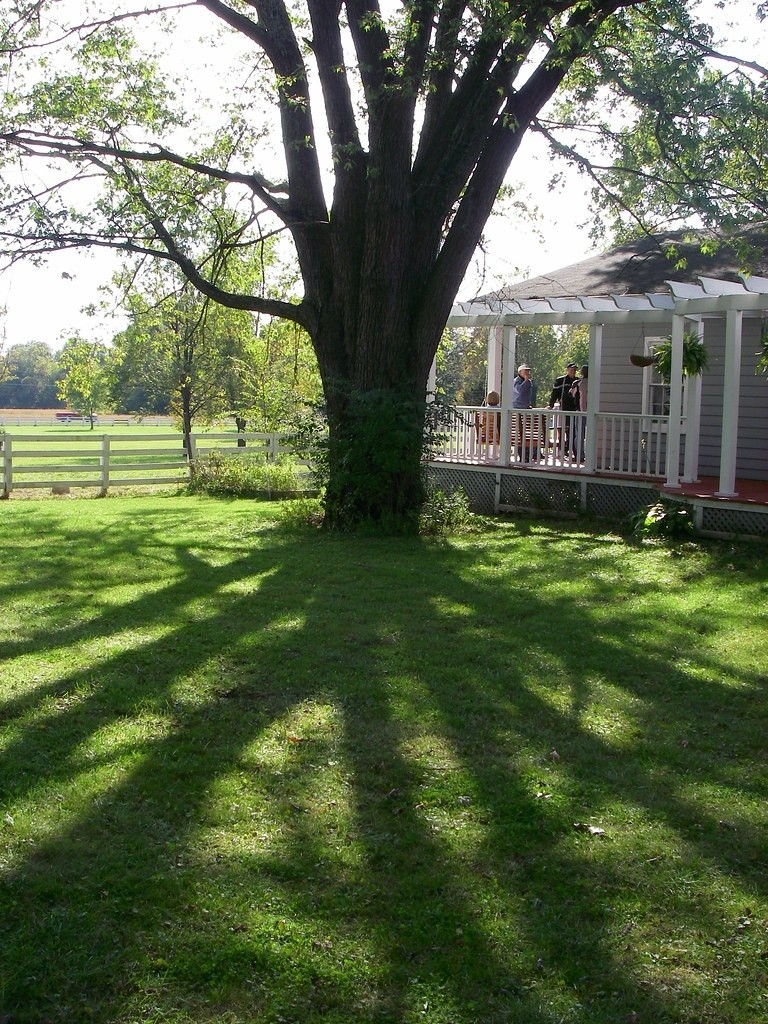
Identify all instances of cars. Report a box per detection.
[56,409,98,422]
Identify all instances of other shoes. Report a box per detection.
[570,457,585,463]
[533,453,550,459]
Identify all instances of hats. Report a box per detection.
[518,364,532,373]
[567,363,578,368]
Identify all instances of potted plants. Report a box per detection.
[648,330,718,384]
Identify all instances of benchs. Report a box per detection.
[475,410,561,448]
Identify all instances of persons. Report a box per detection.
[513,365,545,460]
[547,363,580,457]
[487,391,501,408]
[571,365,588,462]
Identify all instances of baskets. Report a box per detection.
[629,355,655,367]
